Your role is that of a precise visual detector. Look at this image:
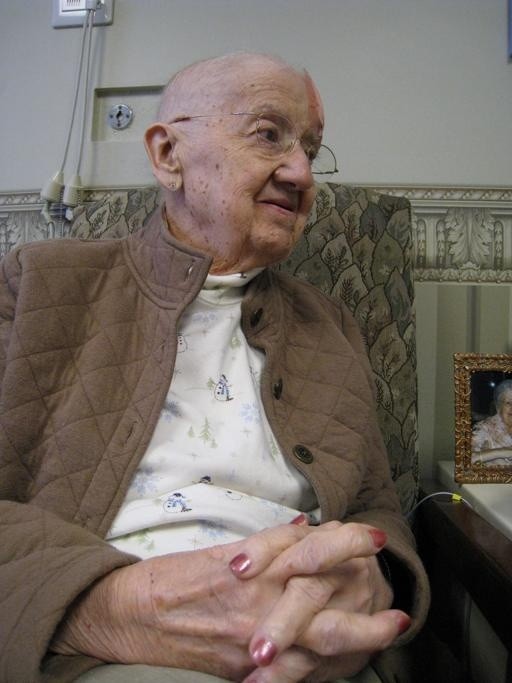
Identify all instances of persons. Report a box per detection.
[0,51,433,681]
[471,378,512,467]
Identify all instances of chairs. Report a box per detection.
[0,180,512,683]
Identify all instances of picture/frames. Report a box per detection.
[454,352,512,485]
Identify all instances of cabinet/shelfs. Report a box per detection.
[432,462,511,682]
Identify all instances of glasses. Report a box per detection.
[166,111,339,185]
